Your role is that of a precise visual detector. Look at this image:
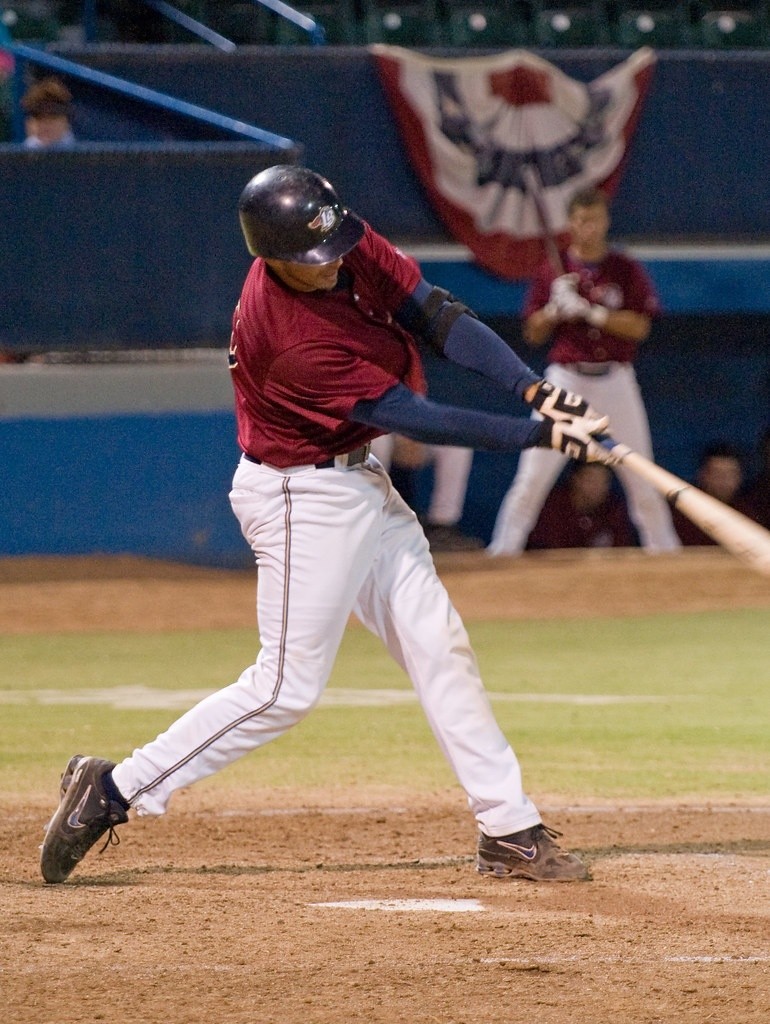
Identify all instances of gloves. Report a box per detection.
[531,382,598,420]
[547,272,609,329]
[537,414,621,466]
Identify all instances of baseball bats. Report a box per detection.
[518,162,566,279]
[589,431,770,582]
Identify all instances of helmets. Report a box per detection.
[239,164,365,264]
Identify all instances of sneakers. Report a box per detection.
[427,524,485,551]
[476,823,590,883]
[40,754,129,884]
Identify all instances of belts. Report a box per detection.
[244,438,370,469]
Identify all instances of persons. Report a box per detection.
[370,431,486,550]
[38,165,615,883]
[486,191,681,553]
[671,455,757,546]
[524,462,636,549]
